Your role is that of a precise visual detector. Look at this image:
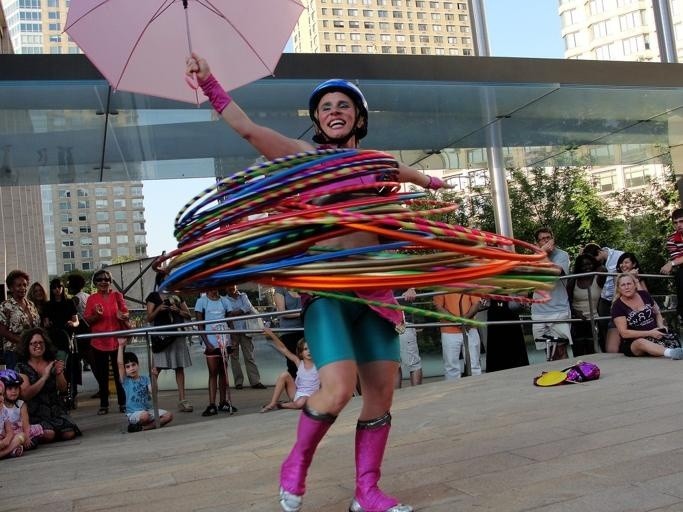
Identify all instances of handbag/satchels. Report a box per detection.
[565,362,599,382]
[121,318,133,343]
[150,323,177,353]
[245,314,265,336]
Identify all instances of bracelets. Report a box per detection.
[425,175,444,190]
[198,74,231,113]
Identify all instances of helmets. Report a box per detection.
[0,369,23,387]
[309,78,368,140]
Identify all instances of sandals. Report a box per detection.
[119,405,126,412]
[98,407,108,414]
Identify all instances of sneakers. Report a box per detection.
[218,400,237,412]
[202,405,217,416]
[236,384,267,389]
[257,400,286,413]
[177,400,193,412]
[128,423,141,432]
[670,348,683,359]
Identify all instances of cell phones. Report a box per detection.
[659,328,666,334]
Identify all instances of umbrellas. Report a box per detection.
[60,0,307,107]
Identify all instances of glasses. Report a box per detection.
[536,236,552,241]
[95,277,109,282]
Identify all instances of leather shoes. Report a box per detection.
[347,497,415,512]
[280,486,304,512]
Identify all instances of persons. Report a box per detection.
[186,53,457,511]
[394,209,682,390]
[0,269,319,458]
